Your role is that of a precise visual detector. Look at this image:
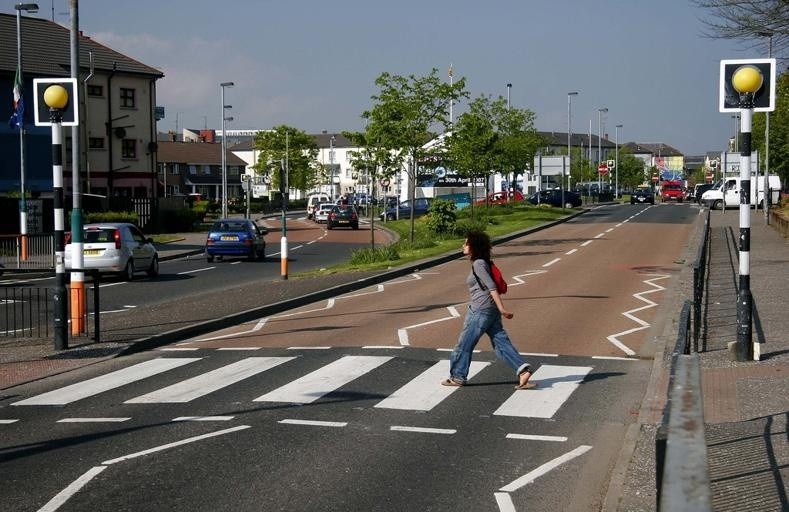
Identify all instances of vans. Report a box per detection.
[306,194,332,219]
[702,174,781,210]
[661,180,683,204]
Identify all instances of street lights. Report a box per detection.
[615,122,624,198]
[447,62,455,128]
[220,80,236,223]
[598,106,607,189]
[505,84,515,110]
[16,2,41,260]
[567,91,578,189]
[33,75,89,347]
[719,58,777,365]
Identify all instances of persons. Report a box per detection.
[442,231,539,391]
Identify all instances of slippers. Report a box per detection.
[515,383,537,390]
[441,378,463,387]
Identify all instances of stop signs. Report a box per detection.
[652,175,658,183]
[599,164,608,175]
[705,174,712,181]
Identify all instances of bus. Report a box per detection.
[576,181,608,190]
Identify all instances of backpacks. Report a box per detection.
[472,257,507,294]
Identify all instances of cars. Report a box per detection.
[63,223,160,283]
[573,186,622,202]
[473,189,525,207]
[631,188,655,205]
[684,182,715,204]
[314,203,359,230]
[380,198,434,221]
[206,218,266,261]
[528,189,582,210]
